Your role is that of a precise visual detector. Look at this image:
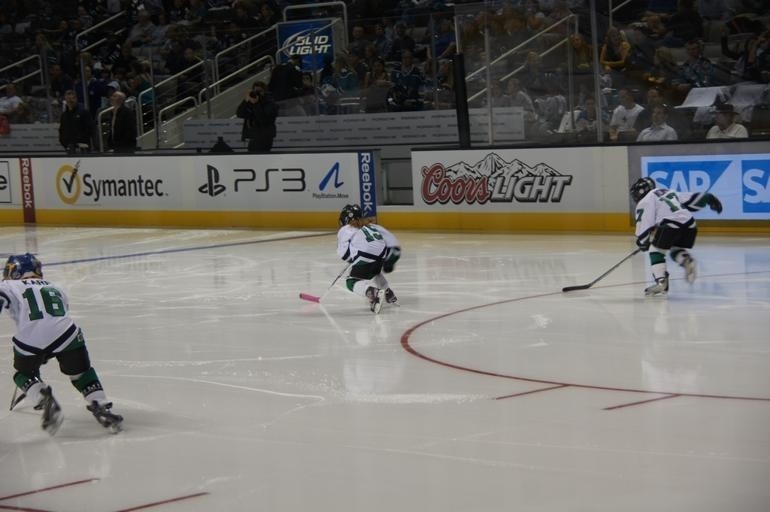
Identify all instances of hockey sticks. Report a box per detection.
[299,262,350,304]
[9,387,26,410]
[562,247,642,291]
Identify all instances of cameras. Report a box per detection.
[249,91,261,98]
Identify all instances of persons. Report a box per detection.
[0,253,123,431]
[337,205,401,313]
[629,176,722,296]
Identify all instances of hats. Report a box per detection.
[710,103,738,114]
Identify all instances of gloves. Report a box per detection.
[708,195,722,213]
[636,240,650,251]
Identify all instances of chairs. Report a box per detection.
[233,0,592,116]
[2,3,277,124]
[535,3,769,140]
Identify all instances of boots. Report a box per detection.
[34,386,60,429]
[645,272,669,293]
[366,286,380,311]
[385,289,397,304]
[680,254,696,283]
[85,400,123,427]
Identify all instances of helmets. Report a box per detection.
[339,204,362,224]
[3,251,42,279]
[630,177,656,202]
